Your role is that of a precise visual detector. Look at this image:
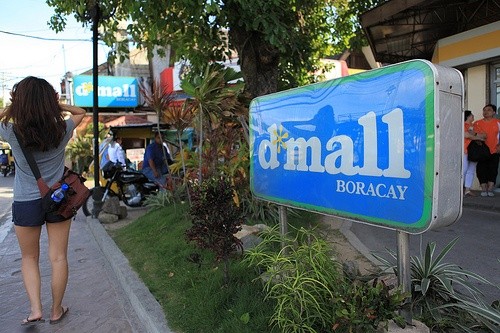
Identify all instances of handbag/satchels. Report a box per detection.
[45,169,91,221]
[468,139,491,163]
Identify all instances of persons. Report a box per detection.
[0,76,86,325]
[141,133,175,192]
[463,110,480,196]
[473,104,500,196]
[0,150,9,172]
[101,129,126,197]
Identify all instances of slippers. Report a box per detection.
[20,317,45,325]
[49,306,68,324]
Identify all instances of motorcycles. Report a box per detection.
[82,159,161,217]
[0,162,8,177]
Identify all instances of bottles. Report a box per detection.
[50,184,68,202]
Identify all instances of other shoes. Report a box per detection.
[481,192,488,196]
[464,189,473,196]
[488,191,495,197]
